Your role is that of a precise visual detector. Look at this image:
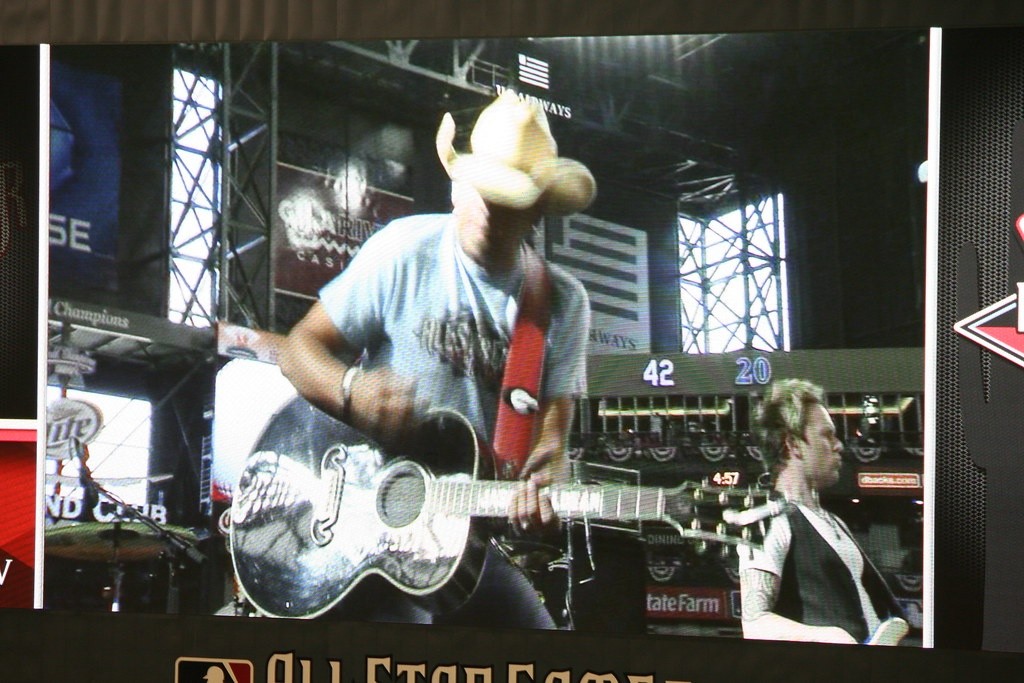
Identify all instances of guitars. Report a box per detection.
[229,375,703,620]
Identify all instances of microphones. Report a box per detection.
[157,530,211,567]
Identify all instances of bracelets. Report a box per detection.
[336,362,357,423]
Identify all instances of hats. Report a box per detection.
[435,92,597,220]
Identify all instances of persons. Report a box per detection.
[273,84,595,628]
[735,378,915,648]
